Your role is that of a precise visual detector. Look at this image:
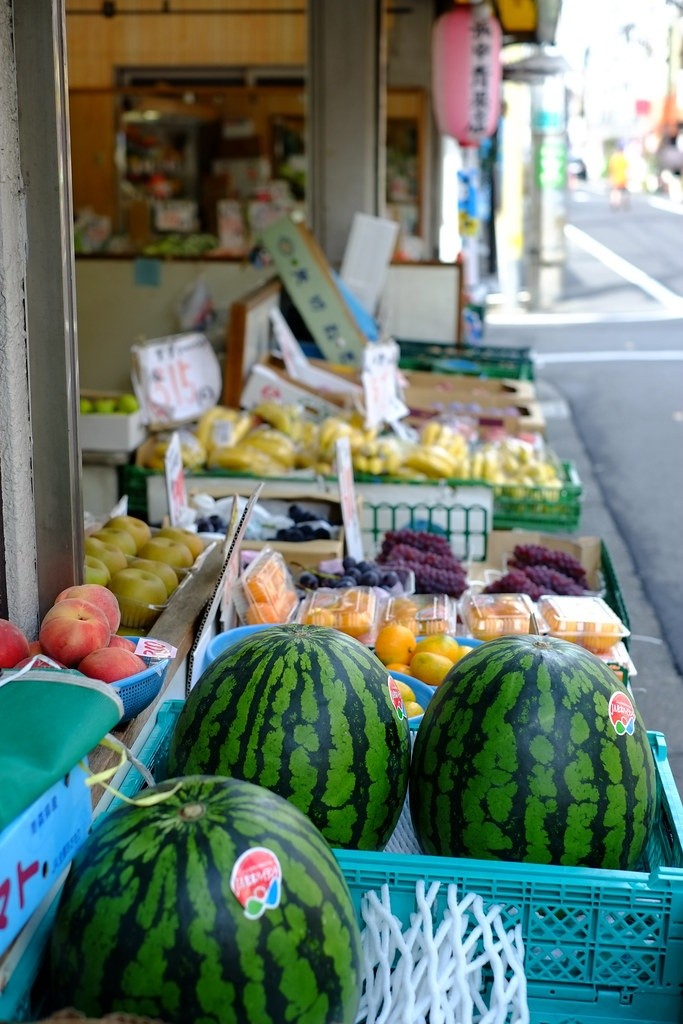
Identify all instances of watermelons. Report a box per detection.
[165,623,411,851]
[409,633,656,872]
[33,773,364,1024]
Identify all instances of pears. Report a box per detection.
[80,393,139,415]
[83,515,204,628]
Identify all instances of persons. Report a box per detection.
[604,138,630,207]
[119,124,306,205]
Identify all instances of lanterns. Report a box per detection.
[431,10,504,156]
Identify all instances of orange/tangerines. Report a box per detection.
[238,559,622,719]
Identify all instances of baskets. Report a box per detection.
[111,340,630,730]
[91,698,683,876]
[0,835,683,1024]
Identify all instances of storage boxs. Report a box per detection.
[164,494,343,570]
[0,696,683,1024]
[79,389,146,454]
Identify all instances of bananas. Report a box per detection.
[140,404,565,515]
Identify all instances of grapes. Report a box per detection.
[376,529,591,602]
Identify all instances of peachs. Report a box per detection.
[0,584,150,685]
